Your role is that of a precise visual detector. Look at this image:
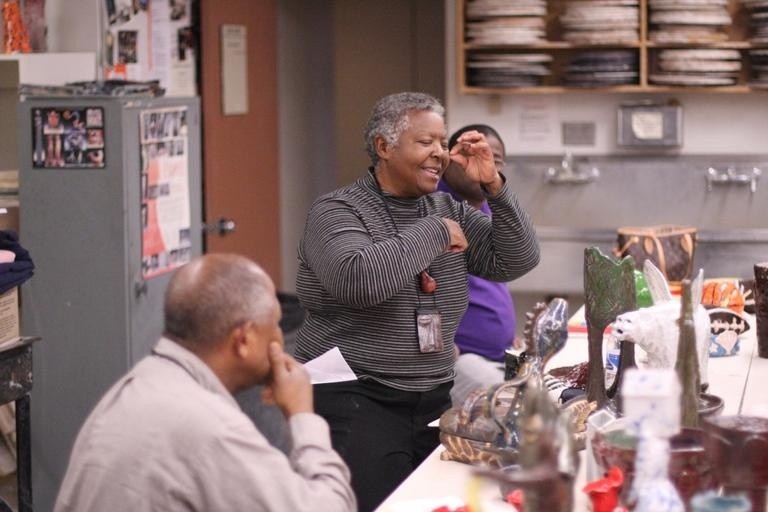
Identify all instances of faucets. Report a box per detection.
[722,160,737,186]
[559,150,573,180]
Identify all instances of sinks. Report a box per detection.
[509,223,619,298]
[696,226,767,276]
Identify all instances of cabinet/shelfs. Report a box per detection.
[20,95,205,510]
[453,1,768,100]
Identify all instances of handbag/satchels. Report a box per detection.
[613,226,698,286]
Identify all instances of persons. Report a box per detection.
[435,124,517,360]
[53,252,360,512]
[294,90,540,512]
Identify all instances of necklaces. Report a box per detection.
[370,167,437,294]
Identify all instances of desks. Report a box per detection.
[371,272,766,509]
